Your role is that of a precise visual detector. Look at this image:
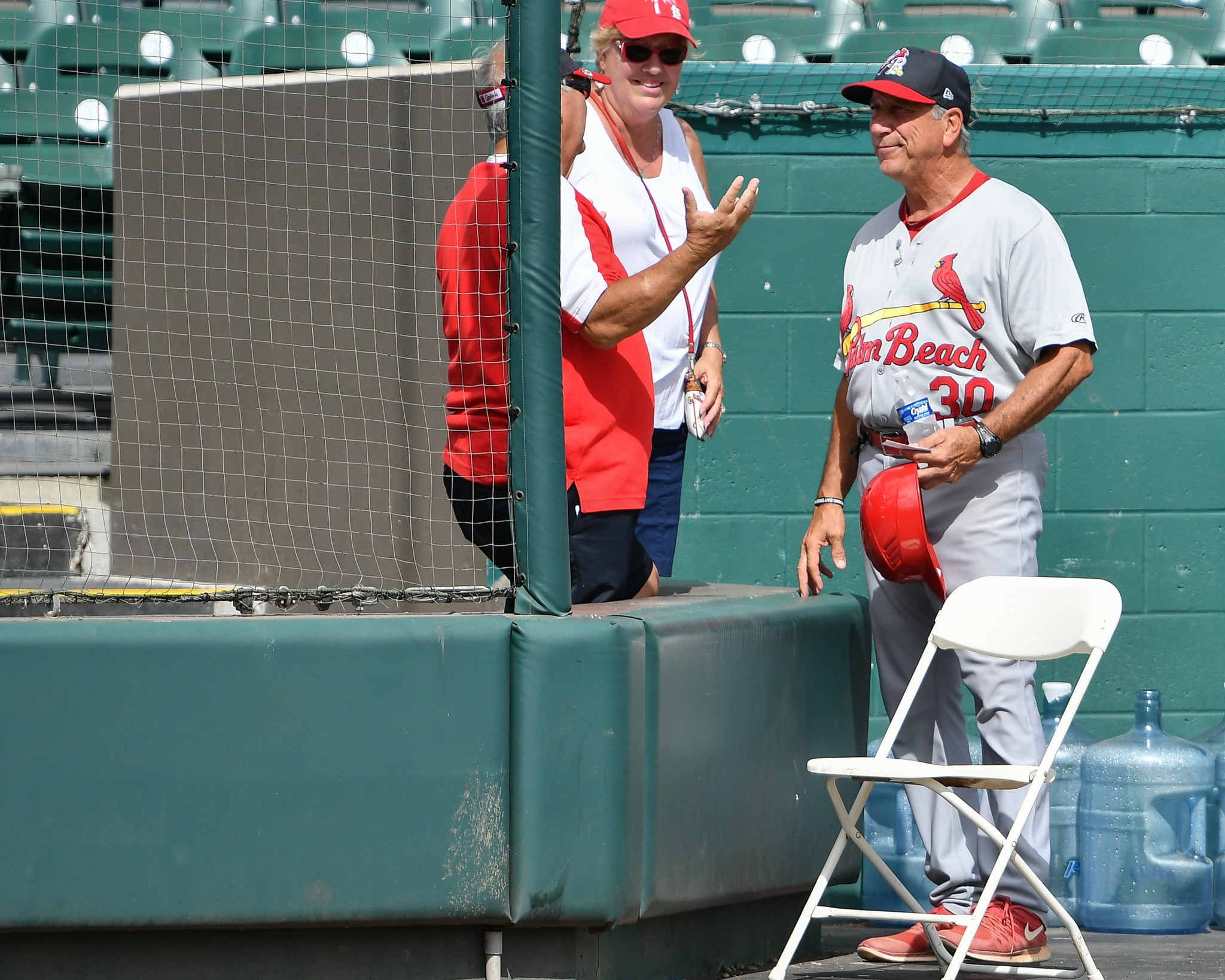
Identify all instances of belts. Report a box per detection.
[859,422,978,456]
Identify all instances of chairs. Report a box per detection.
[766,574,1127,980]
[0,0,1225,356]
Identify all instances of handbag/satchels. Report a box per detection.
[687,382,724,439]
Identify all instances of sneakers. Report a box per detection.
[928,896,1050,963]
[857,899,969,962]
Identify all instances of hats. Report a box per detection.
[477,56,612,107]
[841,47,971,126]
[600,1,698,50]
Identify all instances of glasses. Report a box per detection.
[561,78,591,98]
[614,37,686,66]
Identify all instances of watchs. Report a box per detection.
[971,421,1003,459]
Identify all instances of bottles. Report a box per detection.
[1188,681,1225,932]
[860,733,985,929]
[1075,688,1215,933]
[892,368,941,470]
[1038,682,1100,928]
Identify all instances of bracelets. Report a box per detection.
[813,497,844,508]
[697,342,727,366]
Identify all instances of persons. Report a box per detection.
[795,44,1099,966]
[567,0,727,579]
[434,34,762,612]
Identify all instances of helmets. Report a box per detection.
[860,462,946,603]
